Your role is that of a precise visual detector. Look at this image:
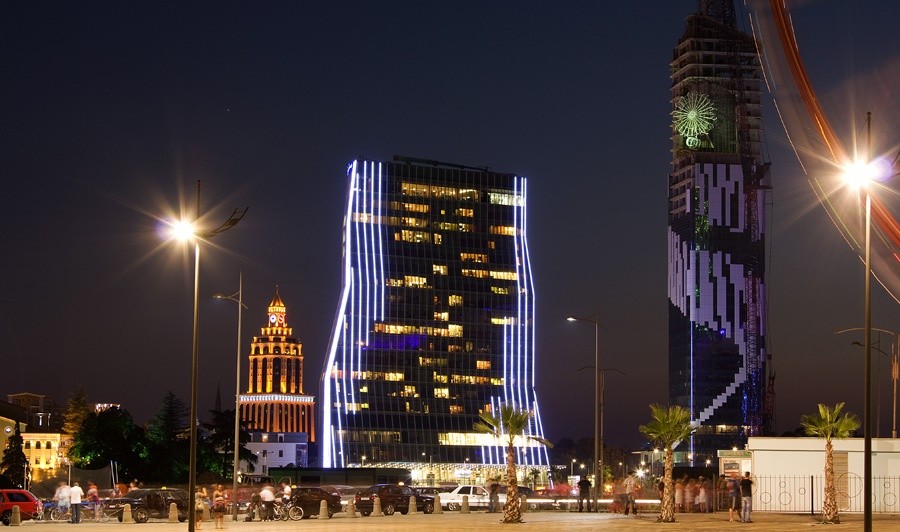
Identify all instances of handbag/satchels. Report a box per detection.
[195,501,204,511]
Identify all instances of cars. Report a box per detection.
[534,490,573,511]
[414,488,444,495]
[354,483,434,516]
[323,486,359,506]
[275,487,342,519]
[438,484,522,511]
[226,491,252,513]
[500,487,546,510]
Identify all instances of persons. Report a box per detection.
[213,485,226,529]
[485,478,502,513]
[658,478,664,502]
[728,474,742,522]
[718,475,726,489]
[54,481,84,524]
[259,482,275,521]
[740,472,754,523]
[674,479,708,513]
[87,483,140,501]
[577,473,592,513]
[195,486,207,530]
[623,472,641,516]
[281,482,291,504]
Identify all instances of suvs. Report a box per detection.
[104,487,189,523]
[0,489,43,526]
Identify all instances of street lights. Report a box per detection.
[848,111,872,532]
[566,316,601,513]
[169,179,203,532]
[212,271,246,523]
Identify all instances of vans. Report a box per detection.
[240,472,291,488]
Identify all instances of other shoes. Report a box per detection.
[740,519,753,523]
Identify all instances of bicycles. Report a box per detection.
[249,490,303,522]
